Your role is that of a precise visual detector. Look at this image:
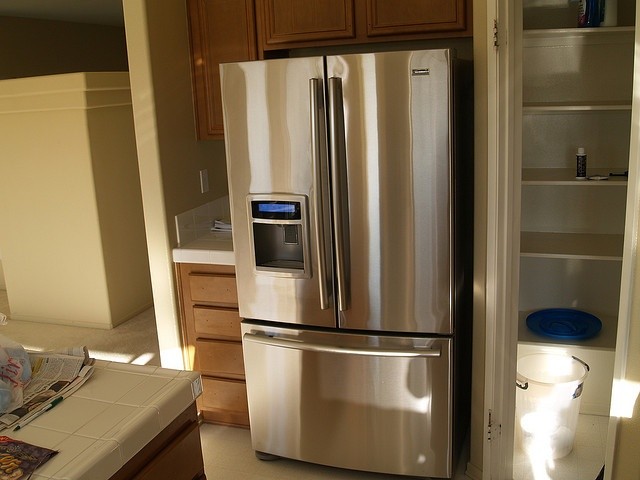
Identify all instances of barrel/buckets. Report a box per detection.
[516,354,591,461]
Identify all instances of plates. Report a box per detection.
[526,308,603,340]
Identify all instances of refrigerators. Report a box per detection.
[218,47,473,480]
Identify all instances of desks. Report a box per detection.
[0,340,205,480]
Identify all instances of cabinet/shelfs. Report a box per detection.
[258,0,472,51]
[501,1,640,480]
[187,0,258,140]
[110,400,206,480]
[179,263,251,429]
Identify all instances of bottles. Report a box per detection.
[599,0,618,27]
[576,147,588,180]
[577,0,600,27]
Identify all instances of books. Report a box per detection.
[210,217,231,232]
